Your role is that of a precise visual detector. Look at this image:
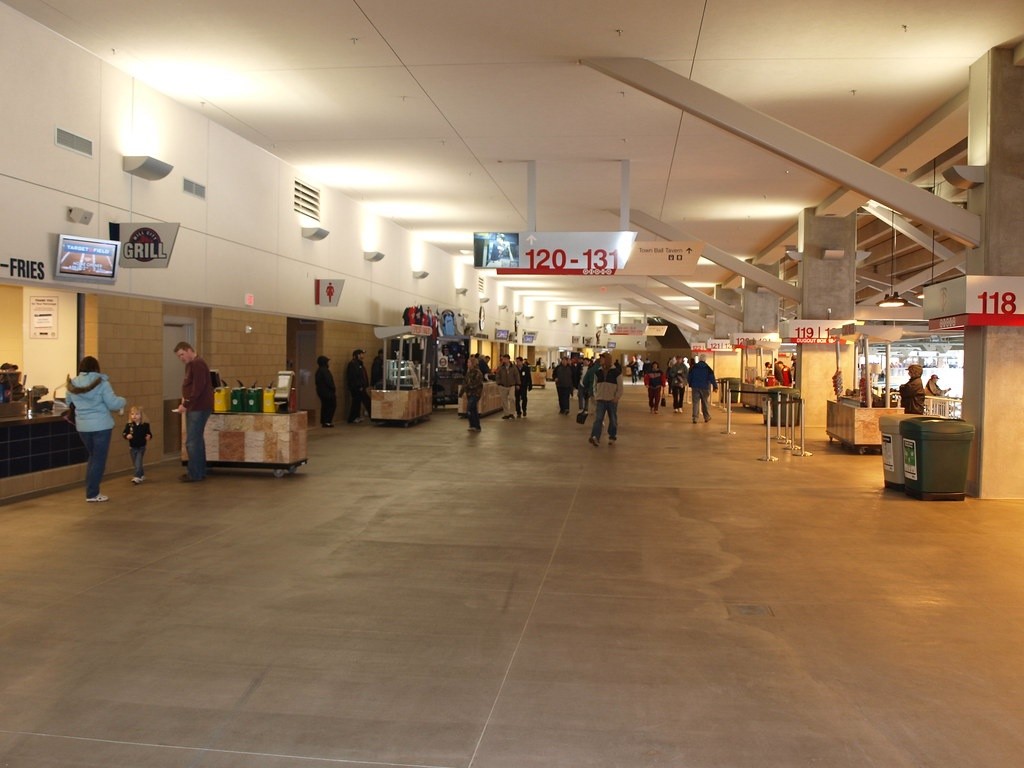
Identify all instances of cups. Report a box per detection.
[275,401,287,413]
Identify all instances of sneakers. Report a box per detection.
[86,493,109,502]
[131,475,146,483]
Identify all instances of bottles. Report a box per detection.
[214,386,275,412]
[288,388,297,412]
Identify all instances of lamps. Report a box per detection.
[123,155,175,181]
[877,211,908,308]
[823,250,845,263]
[943,164,985,190]
[457,288,468,294]
[364,251,385,263]
[515,312,522,315]
[734,287,744,295]
[757,286,770,295]
[414,271,430,279]
[785,250,803,262]
[856,251,872,261]
[526,315,534,319]
[480,297,490,303]
[706,304,736,320]
[302,227,330,241]
[499,305,507,309]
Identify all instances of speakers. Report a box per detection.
[69,207,93,226]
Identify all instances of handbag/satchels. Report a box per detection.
[661,393,666,407]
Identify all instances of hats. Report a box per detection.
[791,355,797,361]
[355,349,367,354]
[0,363,17,371]
[905,365,922,370]
[931,375,939,379]
[765,362,769,368]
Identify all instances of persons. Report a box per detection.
[523,359,530,373]
[626,354,644,383]
[765,358,792,387]
[536,358,542,372]
[515,357,532,417]
[925,375,951,396]
[175,341,214,483]
[666,356,690,413]
[471,354,492,383]
[459,358,483,431]
[315,356,337,428]
[900,365,925,415]
[589,353,623,448]
[496,354,521,419]
[614,359,621,374]
[644,361,666,413]
[66,356,126,502]
[688,353,718,423]
[443,344,454,368]
[349,350,381,423]
[371,350,396,390]
[553,356,595,416]
[0,363,18,405]
[123,405,152,484]
[643,357,653,381]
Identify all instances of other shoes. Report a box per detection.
[559,409,569,415]
[674,409,678,413]
[502,416,509,419]
[523,411,526,416]
[353,417,363,423]
[679,408,682,412]
[322,423,334,427]
[705,417,711,422]
[509,414,515,419]
[182,474,205,482]
[589,436,600,447]
[467,426,481,431]
[608,440,615,446]
[650,406,658,414]
[517,414,521,419]
[693,420,697,424]
[578,408,588,414]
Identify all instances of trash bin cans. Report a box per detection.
[878,414,975,501]
[720,377,740,403]
[761,386,802,426]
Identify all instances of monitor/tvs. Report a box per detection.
[54,234,121,282]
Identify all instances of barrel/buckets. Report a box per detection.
[764,376,776,387]
